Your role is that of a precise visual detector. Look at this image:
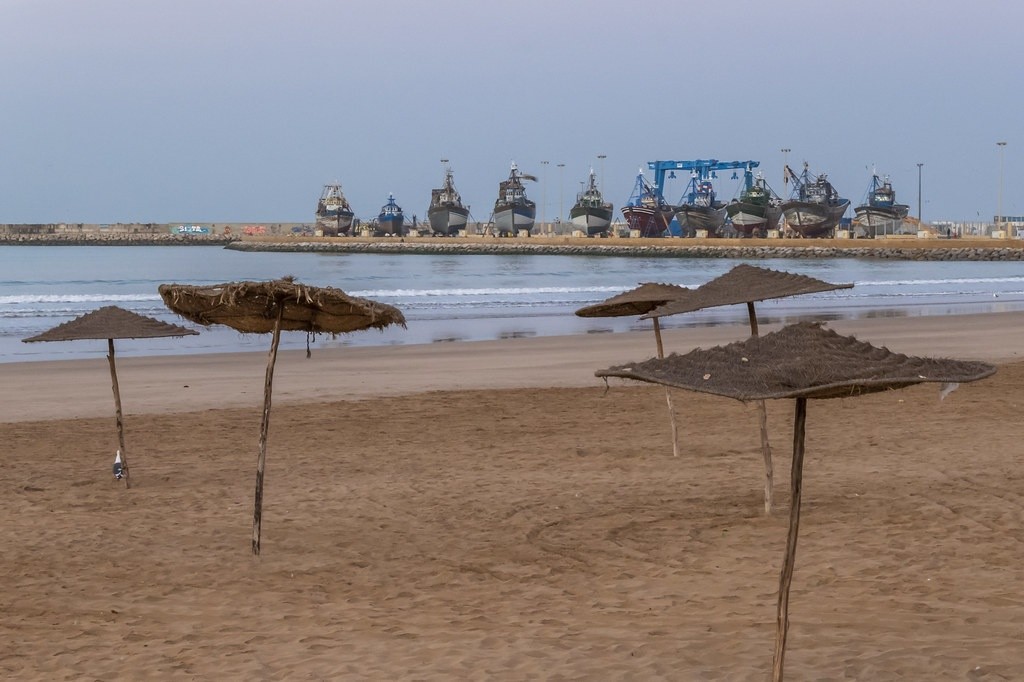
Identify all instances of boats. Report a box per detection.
[427,151,471,234]
[727,170,784,234]
[620,167,675,237]
[854,162,910,235]
[493,157,538,237]
[366,192,406,236]
[567,166,614,235]
[674,166,729,235]
[314,179,354,236]
[779,157,852,238]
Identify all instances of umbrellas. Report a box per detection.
[22,306,199,490]
[595,320,998,682]
[638,263,854,517]
[159,276,406,558]
[575,282,692,456]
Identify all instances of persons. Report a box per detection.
[947,228,950,239]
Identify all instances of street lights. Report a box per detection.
[780,148,791,202]
[996,142,1007,230]
[917,163,923,220]
